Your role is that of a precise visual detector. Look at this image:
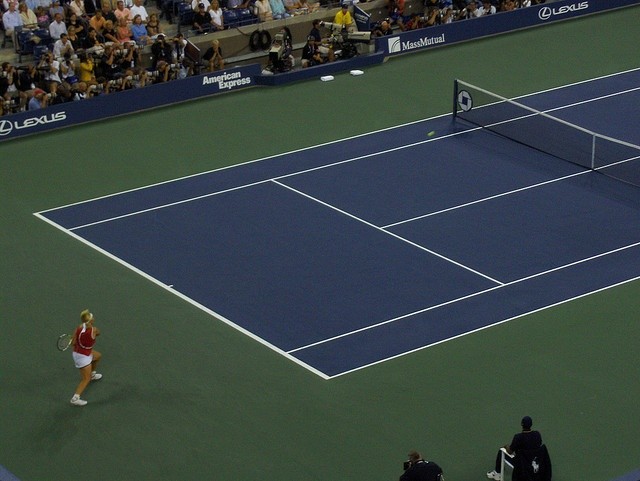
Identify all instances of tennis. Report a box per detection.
[428,132,432,137]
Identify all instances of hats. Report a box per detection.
[198,3,204,7]
[340,4,348,9]
[307,35,315,42]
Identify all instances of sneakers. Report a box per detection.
[70,396,88,406]
[487,470,503,480]
[91,374,103,380]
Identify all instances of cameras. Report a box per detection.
[404,460,412,470]
[269,33,291,72]
[44,54,50,63]
[320,21,371,59]
[138,45,144,49]
[370,21,382,34]
[35,66,50,72]
[11,66,29,70]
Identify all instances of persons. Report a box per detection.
[171,33,187,62]
[36,6,49,24]
[515,1,531,9]
[388,1,407,32]
[67,13,85,36]
[487,415,541,480]
[253,0,273,22]
[4,0,19,11]
[114,1,130,22]
[399,450,442,481]
[500,0,515,12]
[55,82,72,107]
[66,26,78,45]
[60,53,77,82]
[441,8,456,24]
[309,19,334,63]
[19,64,41,98]
[194,0,211,32]
[0,62,27,111]
[274,0,290,20]
[479,2,497,16]
[192,1,211,13]
[18,2,39,31]
[69,309,103,405]
[157,61,170,82]
[147,14,166,39]
[2,2,22,55]
[427,7,441,28]
[278,25,294,68]
[28,89,56,110]
[90,10,106,30]
[50,0,65,21]
[49,13,68,39]
[99,45,115,77]
[133,70,148,90]
[228,0,251,15]
[375,20,393,37]
[53,33,74,59]
[301,35,323,67]
[131,14,153,44]
[103,20,120,46]
[151,34,171,71]
[299,0,320,13]
[84,27,104,55]
[211,0,224,30]
[73,82,96,101]
[80,53,94,82]
[96,77,110,95]
[38,50,61,93]
[116,17,135,44]
[333,4,353,28]
[202,40,224,72]
[69,1,84,16]
[285,0,299,14]
[123,42,141,75]
[131,0,148,21]
[113,72,129,93]
[462,2,481,19]
[407,13,420,30]
[176,59,197,79]
[101,2,118,28]
